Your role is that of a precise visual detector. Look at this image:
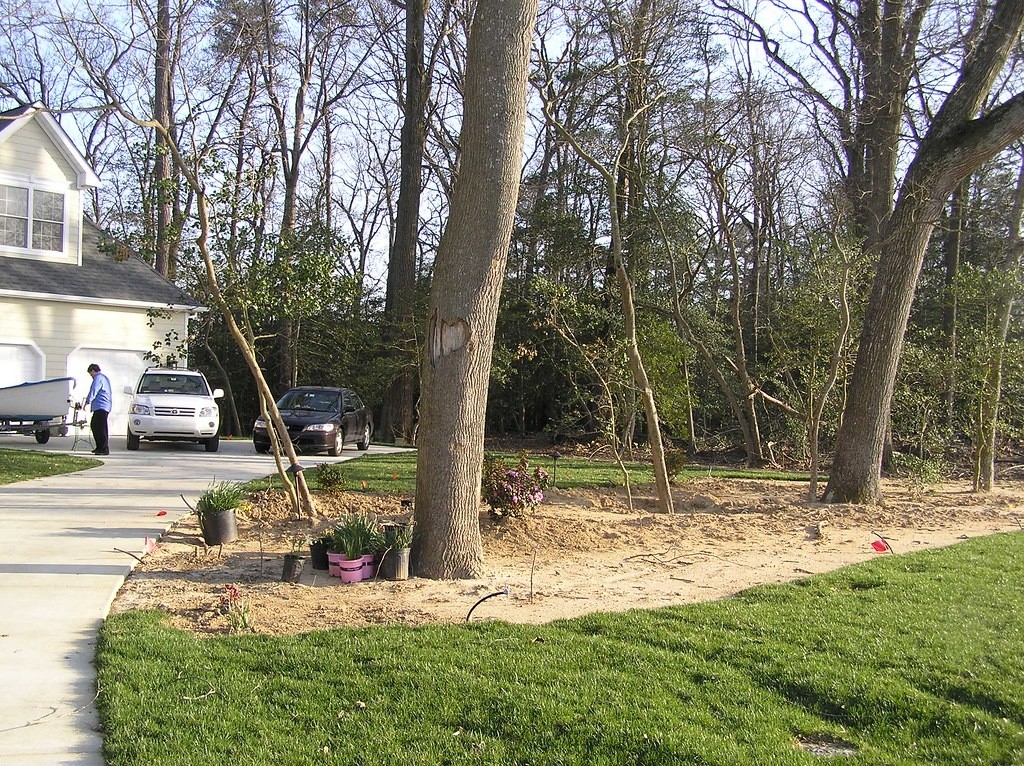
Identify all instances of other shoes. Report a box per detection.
[92,449,109,455]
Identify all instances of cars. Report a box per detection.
[252,385,374,457]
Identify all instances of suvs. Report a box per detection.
[123,366,225,453]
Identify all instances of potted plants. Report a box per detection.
[309,512,411,582]
[282,537,306,583]
[193,476,244,544]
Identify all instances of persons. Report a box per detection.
[83,364,112,455]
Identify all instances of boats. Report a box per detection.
[0,376,77,422]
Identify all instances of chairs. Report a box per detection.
[148,381,160,391]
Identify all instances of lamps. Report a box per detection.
[550,451,561,458]
